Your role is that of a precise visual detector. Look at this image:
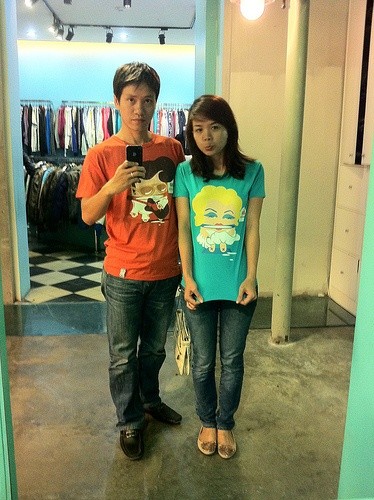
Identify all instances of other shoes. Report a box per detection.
[218,429,236,459]
[147,401,182,425]
[198,425,217,455]
[120,430,145,460]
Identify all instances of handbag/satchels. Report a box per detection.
[173,291,191,375]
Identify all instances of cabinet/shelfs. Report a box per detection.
[328,0,374,317]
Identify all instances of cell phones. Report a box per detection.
[126,146,143,178]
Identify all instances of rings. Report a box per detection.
[130,172,135,178]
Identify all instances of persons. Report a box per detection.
[75,63,186,461]
[174,95,267,459]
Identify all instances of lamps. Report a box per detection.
[49,13,64,40]
[105,27,113,43]
[157,28,167,44]
[65,25,74,41]
[124,0,131,8]
[240,0,286,20]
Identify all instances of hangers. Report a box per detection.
[61,101,118,110]
[156,103,193,111]
[34,157,83,167]
[20,100,54,107]
